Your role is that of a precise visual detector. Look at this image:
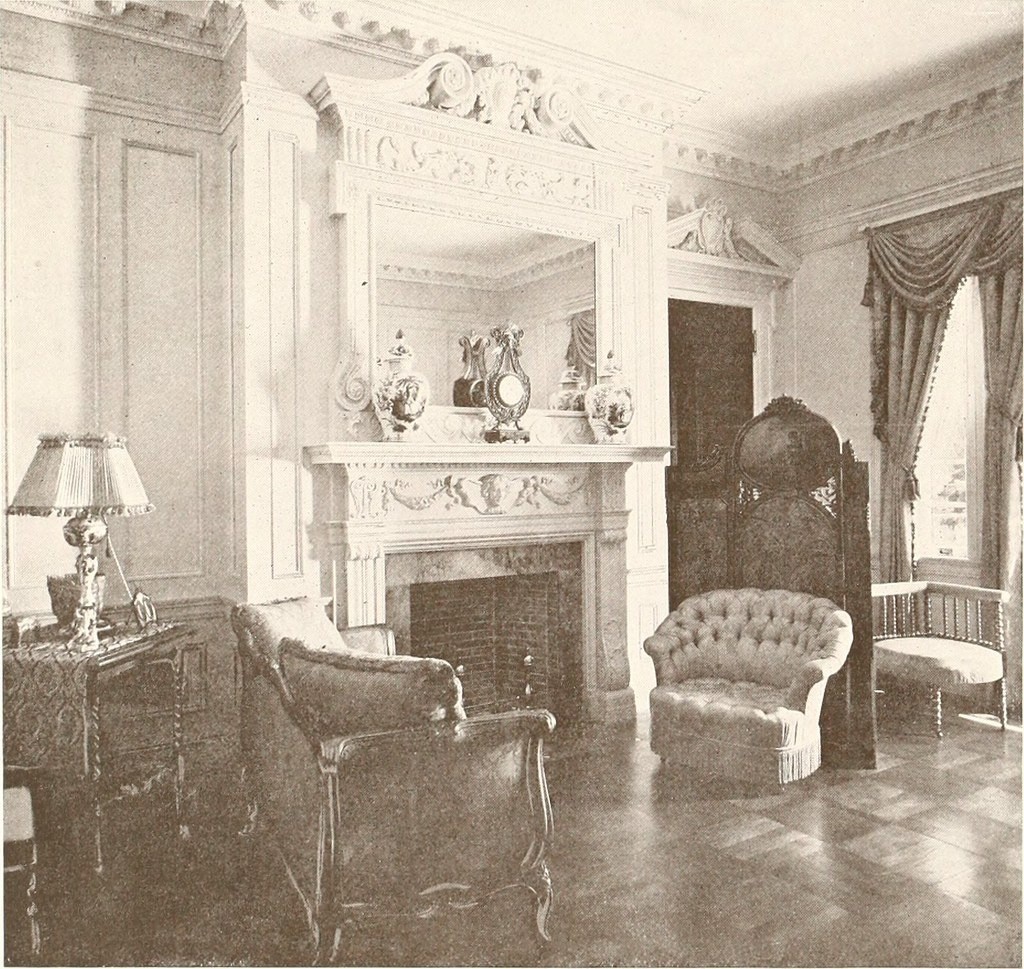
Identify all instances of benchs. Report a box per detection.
[239,626,559,966]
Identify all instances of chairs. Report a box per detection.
[871,581,1009,739]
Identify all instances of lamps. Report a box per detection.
[5,433,154,650]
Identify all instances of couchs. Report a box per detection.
[643,585,852,790]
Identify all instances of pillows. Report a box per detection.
[277,636,467,733]
[231,596,349,660]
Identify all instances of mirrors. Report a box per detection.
[367,195,612,420]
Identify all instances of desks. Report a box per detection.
[3,620,198,883]
